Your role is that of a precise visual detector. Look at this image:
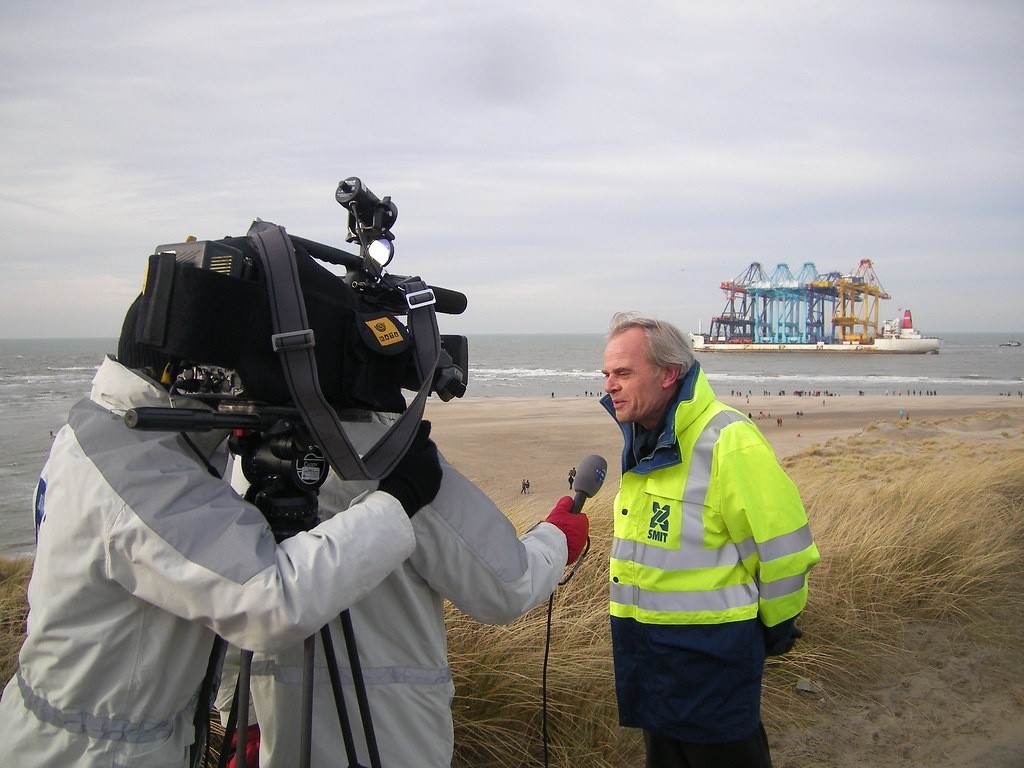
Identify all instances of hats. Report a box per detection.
[118,292,214,370]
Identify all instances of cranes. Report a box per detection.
[709,259,891,344]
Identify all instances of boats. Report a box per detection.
[688,309,942,355]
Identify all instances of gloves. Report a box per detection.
[377,420,442,520]
[764,623,805,658]
[224,724,260,768]
[545,494,588,567]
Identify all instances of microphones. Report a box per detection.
[381,286,467,314]
[571,455,607,514]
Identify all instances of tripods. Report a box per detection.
[123,405,382,768]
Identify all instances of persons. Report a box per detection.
[748,411,772,421]
[599,318,820,768]
[823,400,825,406]
[795,410,804,418]
[49,429,54,438]
[519,479,530,494]
[763,390,786,396]
[731,389,752,403]
[859,389,865,396]
[0,290,443,768]
[212,381,589,768]
[776,418,785,428]
[567,467,576,490]
[575,391,603,398]
[898,408,909,420]
[1000,390,1024,397]
[793,389,841,397]
[551,392,555,397]
[885,388,937,396]
